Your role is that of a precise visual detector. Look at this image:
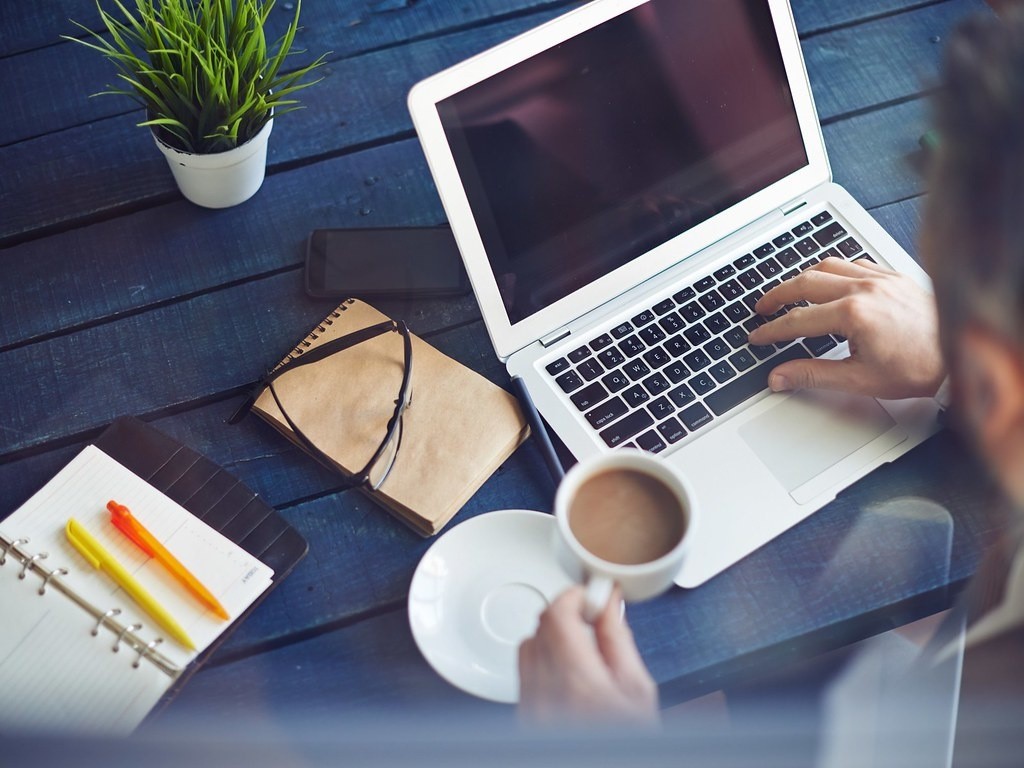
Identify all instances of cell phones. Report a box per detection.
[307,228,472,297]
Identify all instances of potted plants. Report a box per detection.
[59,1,335,210]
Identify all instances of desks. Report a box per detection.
[0,0,1024,723]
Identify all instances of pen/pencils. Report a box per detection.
[64,519,196,653]
[106,500,231,620]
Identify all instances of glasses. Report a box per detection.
[229,320,413,511]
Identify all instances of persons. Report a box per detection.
[517,1,1024,746]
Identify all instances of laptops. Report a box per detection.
[407,0,954,592]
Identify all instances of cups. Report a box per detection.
[554,447,695,620]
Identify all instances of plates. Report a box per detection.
[408,510,625,703]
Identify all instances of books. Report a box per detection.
[249,299,532,537]
[1,415,310,734]
